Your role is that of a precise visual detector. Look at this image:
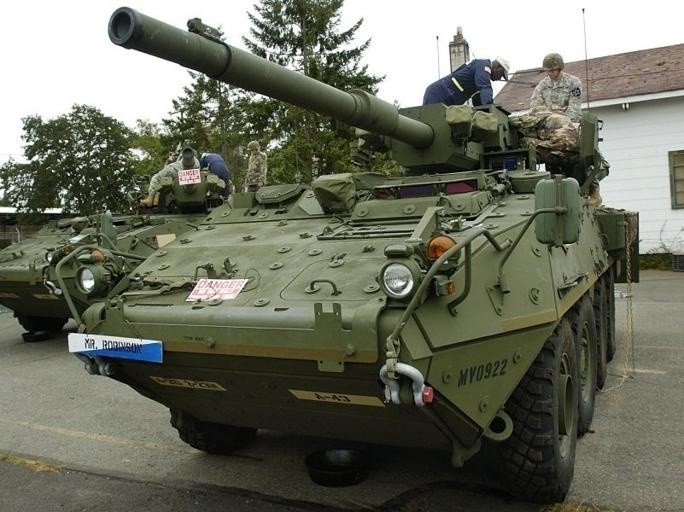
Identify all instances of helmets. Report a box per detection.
[247,140,260,151]
[542,53,564,72]
[530,106,551,114]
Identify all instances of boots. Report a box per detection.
[141,192,154,206]
[586,189,603,205]
[153,191,160,206]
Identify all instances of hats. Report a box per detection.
[496,57,511,82]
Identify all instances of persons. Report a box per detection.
[586,149,610,205]
[508,106,578,171]
[200,153,230,197]
[244,141,267,192]
[423,57,511,105]
[140,161,185,208]
[530,53,584,123]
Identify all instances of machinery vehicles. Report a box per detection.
[0,147,234,344]
[70,5,646,506]
